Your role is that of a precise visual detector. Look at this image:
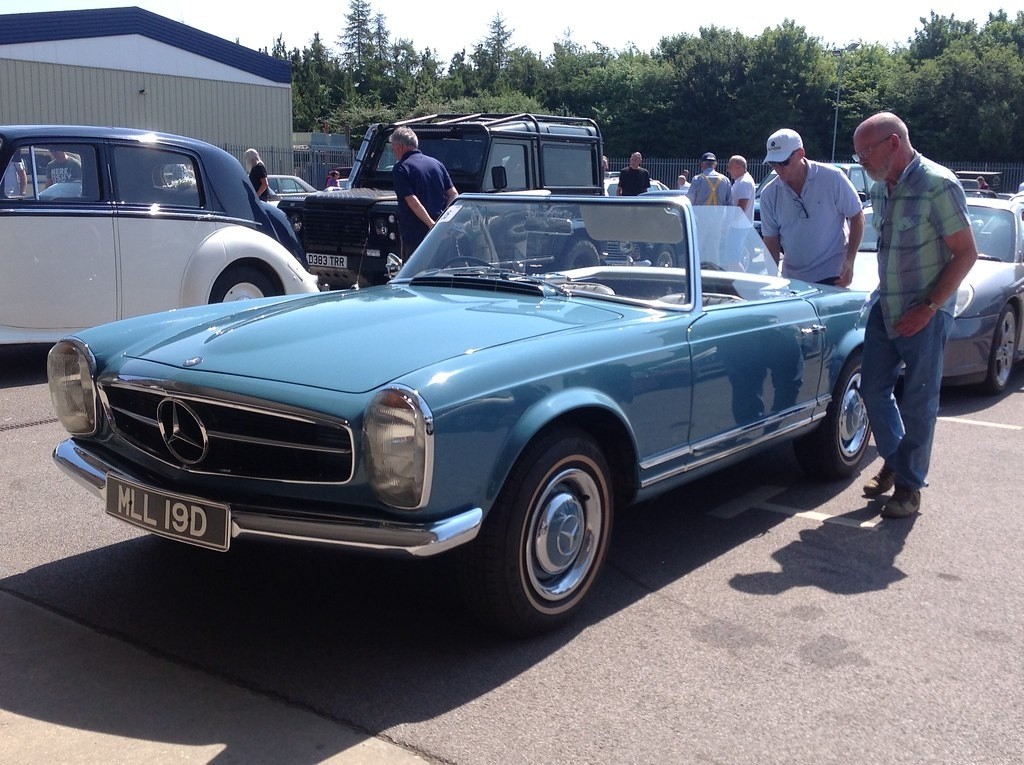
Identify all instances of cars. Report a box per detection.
[824,199,1024,393]
[954,171,1024,204]
[0,121,322,345]
[562,187,764,268]
[19,149,83,197]
[604,170,670,195]
[183,174,320,209]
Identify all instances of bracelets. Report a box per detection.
[19,192,25,195]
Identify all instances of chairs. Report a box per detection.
[658,292,747,306]
[556,282,616,296]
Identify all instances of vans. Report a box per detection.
[749,161,876,244]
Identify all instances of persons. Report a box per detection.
[686,152,733,271]
[390,126,458,266]
[760,129,866,287]
[616,152,650,196]
[245,149,269,202]
[852,113,977,518]
[45,149,82,187]
[722,307,803,424]
[724,155,755,272]
[977,176,988,189]
[678,170,691,190]
[4,158,27,196]
[327,171,340,190]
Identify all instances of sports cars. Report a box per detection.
[45,195,877,634]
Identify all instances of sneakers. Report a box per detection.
[863,463,895,497]
[881,489,920,517]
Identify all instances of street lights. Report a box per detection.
[830,42,859,165]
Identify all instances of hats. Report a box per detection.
[701,153,716,163]
[762,129,804,164]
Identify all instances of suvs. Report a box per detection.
[301,112,601,290]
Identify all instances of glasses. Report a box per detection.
[792,196,809,219]
[852,134,902,162]
[767,148,801,167]
[873,218,886,252]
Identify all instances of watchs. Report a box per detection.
[925,298,939,312]
